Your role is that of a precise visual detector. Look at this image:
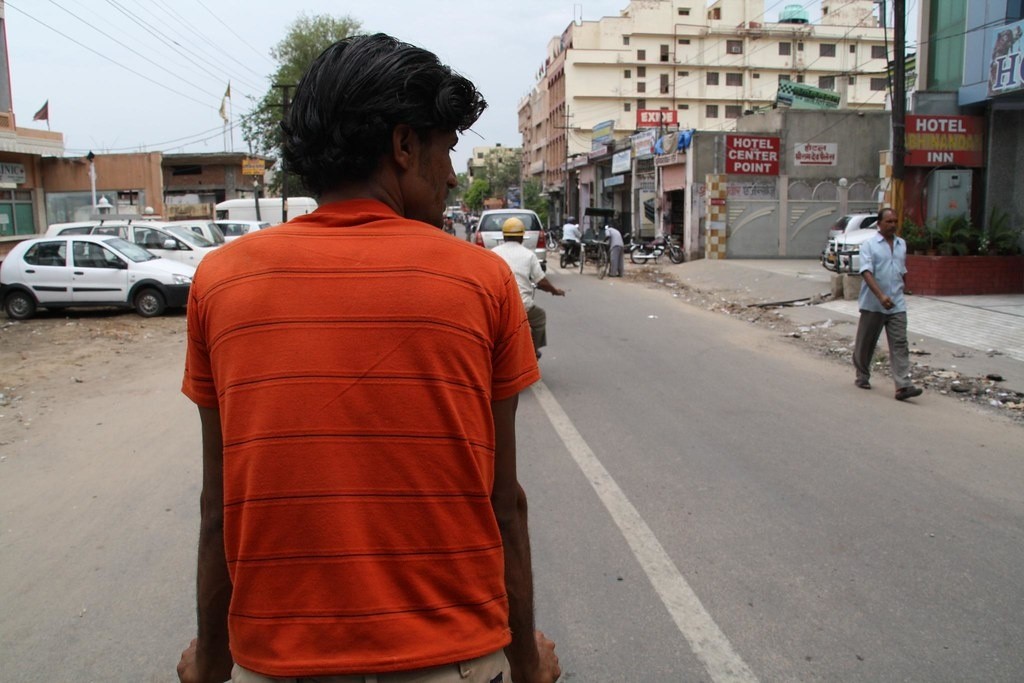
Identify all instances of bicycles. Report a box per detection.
[560,242,580,274]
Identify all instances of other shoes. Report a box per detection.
[895,386,922,399]
[855,380,871,389]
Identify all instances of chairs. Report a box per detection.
[233,225,244,235]
[136,232,164,251]
[91,244,108,267]
[73,245,90,267]
[223,226,235,235]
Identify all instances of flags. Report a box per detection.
[34,103,47,119]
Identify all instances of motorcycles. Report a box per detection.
[624,232,685,264]
[543,225,558,251]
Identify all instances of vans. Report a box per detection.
[476,209,549,272]
[213,196,318,226]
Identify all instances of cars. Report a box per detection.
[820,211,882,274]
[0,219,272,320]
[444,205,465,219]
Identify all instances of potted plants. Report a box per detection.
[900,209,1020,258]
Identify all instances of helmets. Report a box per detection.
[567,216,577,224]
[502,217,525,236]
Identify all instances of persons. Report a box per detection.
[604,224,624,277]
[853,208,922,400]
[444,218,453,231]
[562,216,582,260]
[177,32,562,683]
[487,217,564,359]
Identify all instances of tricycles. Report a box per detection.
[584,240,611,279]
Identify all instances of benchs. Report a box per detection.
[34,247,65,265]
